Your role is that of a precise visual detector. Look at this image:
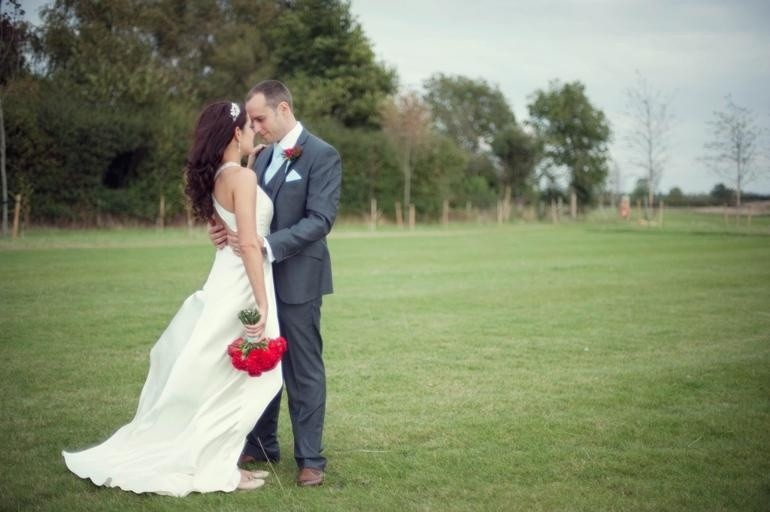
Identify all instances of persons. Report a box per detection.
[208,82,341,487]
[63,100,284,498]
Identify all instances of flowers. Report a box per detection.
[225,308,290,377]
[279,144,303,162]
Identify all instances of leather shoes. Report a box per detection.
[237,469,269,490]
[297,467,324,487]
[238,453,257,464]
